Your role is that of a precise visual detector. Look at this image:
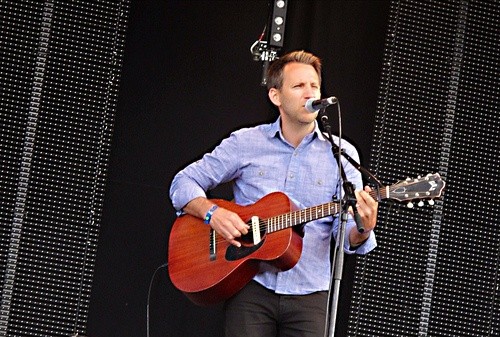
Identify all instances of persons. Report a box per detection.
[169,49,379,336]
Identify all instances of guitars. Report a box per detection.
[168,173,446,308]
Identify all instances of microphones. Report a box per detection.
[305,96,338,113]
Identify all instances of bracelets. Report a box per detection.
[203,204,218,224]
